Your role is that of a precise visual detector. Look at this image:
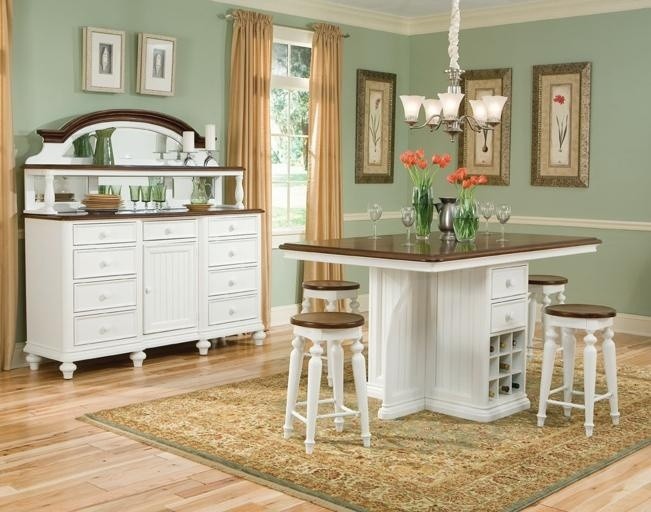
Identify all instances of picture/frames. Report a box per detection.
[80,25,125,94]
[356,67,396,185]
[136,31,177,96]
[455,67,512,188]
[529,61,590,189]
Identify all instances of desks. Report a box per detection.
[275,226,612,425]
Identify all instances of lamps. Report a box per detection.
[398,1,508,154]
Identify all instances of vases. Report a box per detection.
[450,191,481,241]
[412,184,431,241]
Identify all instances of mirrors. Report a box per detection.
[62,126,200,167]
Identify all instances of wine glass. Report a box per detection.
[367,201,385,240]
[494,202,511,242]
[129,184,169,214]
[478,199,495,236]
[401,206,418,246]
[99,184,122,195]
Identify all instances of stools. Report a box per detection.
[283,313,374,454]
[528,275,569,353]
[538,303,624,440]
[299,281,365,389]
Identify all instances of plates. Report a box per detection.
[81,193,124,209]
[39,193,76,202]
[182,204,214,211]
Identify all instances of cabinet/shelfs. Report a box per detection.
[19,221,139,362]
[490,268,531,414]
[200,210,260,335]
[143,216,199,345]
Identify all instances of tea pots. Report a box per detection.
[73,132,94,157]
[435,198,459,242]
[88,126,117,167]
[191,179,214,203]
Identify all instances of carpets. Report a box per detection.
[72,365,650,512]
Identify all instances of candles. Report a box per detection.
[204,123,215,148]
[182,131,193,152]
[152,132,167,153]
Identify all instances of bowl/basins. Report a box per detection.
[50,203,84,213]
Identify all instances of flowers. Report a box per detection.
[446,167,490,234]
[400,150,453,230]
